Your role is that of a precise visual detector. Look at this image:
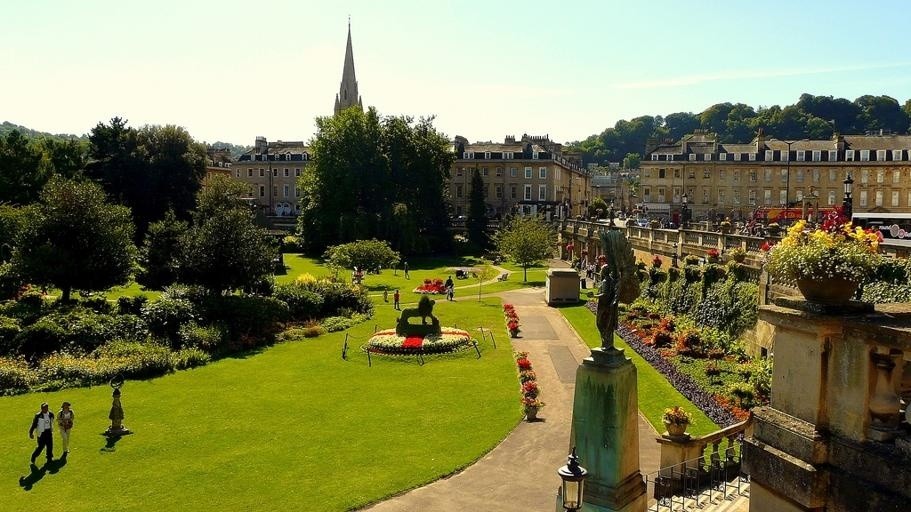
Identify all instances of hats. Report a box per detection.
[61,402,71,408]
[41,403,48,409]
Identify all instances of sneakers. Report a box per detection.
[31,455,36,462]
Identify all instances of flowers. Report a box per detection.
[513,349,540,425]
[501,302,523,340]
[634,246,748,270]
[758,205,884,285]
[662,406,690,423]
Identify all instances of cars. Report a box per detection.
[635,218,650,228]
[619,213,627,220]
[616,210,622,217]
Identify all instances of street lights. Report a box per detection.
[462,168,467,218]
[610,189,616,227]
[681,192,689,223]
[557,447,589,512]
[266,169,275,214]
[842,174,855,221]
[770,137,810,224]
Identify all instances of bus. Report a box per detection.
[754,207,840,228]
[850,212,911,240]
[233,198,264,215]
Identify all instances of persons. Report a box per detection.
[445,275,453,294]
[29,402,55,464]
[405,261,410,280]
[57,401,75,453]
[394,289,401,310]
[586,261,593,278]
[384,288,389,302]
[587,264,618,351]
[446,284,454,301]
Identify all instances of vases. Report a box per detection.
[663,421,691,435]
[795,272,859,303]
